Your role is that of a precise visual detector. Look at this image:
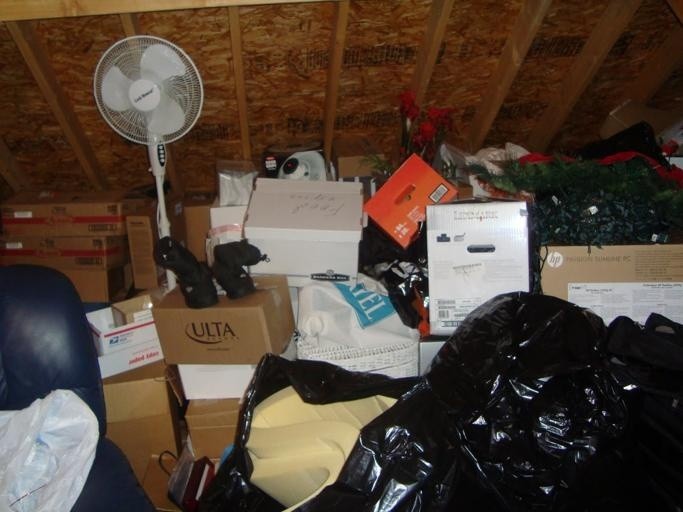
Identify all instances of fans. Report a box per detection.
[94,36,204,305]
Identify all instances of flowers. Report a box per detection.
[458,139,683,255]
[354,91,468,177]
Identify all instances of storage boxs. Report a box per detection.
[0,177,683,512]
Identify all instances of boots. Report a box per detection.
[212,241,262,298]
[152,236,217,308]
